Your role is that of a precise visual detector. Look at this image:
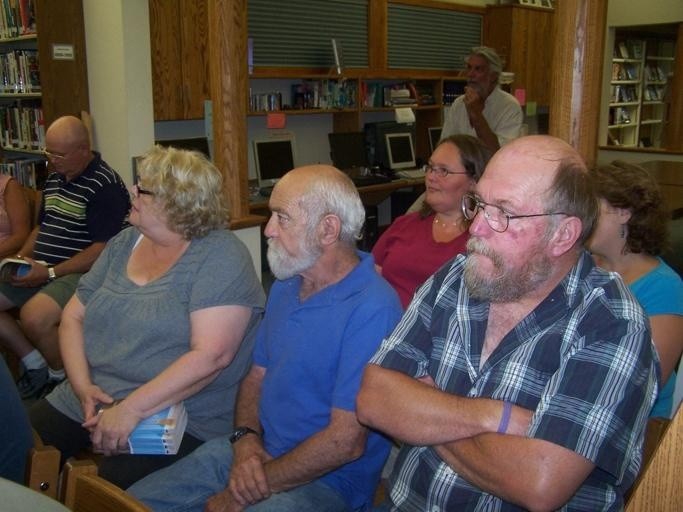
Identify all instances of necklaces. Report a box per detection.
[431,215,465,231]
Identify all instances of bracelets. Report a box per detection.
[498,402,515,435]
[15,253,22,259]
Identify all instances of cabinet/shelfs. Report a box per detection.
[597,27,682,152]
[0,0,90,192]
[148,0,556,122]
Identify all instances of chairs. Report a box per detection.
[25,423,62,502]
[60,459,155,512]
[622,401,683,512]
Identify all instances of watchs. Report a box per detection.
[225,427,260,443]
[47,266,58,283]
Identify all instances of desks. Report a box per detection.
[244,162,426,253]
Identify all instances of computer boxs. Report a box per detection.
[389,191,425,223]
[356,205,378,252]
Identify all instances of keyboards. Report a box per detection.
[398,169,424,178]
[350,176,391,187]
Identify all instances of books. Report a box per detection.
[0,0,47,193]
[250,80,467,112]
[0,258,48,285]
[608,34,665,147]
[89,398,189,457]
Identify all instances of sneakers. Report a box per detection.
[40,376,69,402]
[15,365,50,398]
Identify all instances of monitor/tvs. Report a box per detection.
[253,134,298,187]
[384,132,416,173]
[155,137,211,162]
[427,127,442,156]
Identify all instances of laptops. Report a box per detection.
[327,131,391,178]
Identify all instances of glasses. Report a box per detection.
[460,191,570,233]
[43,145,82,161]
[421,162,475,177]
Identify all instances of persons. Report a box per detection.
[0,358,33,482]
[29,145,266,490]
[0,114,131,400]
[437,46,523,173]
[122,161,405,512]
[1,171,31,266]
[369,136,494,311]
[355,134,657,512]
[588,160,681,497]
